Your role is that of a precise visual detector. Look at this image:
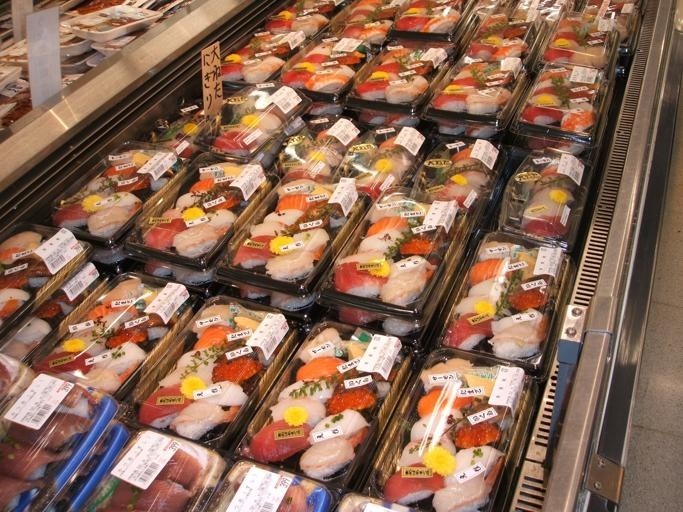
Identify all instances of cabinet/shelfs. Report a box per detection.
[0,0,683,512]
